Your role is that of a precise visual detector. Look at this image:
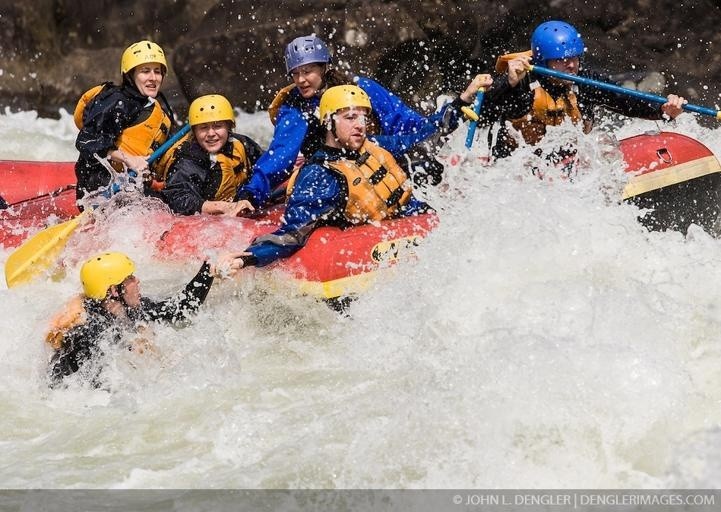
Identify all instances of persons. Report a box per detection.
[46,251,253,391]
[74,40,171,213]
[476,19,688,179]
[215,73,495,279]
[222,36,435,219]
[162,95,266,216]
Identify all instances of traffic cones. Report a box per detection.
[1,133,721,319]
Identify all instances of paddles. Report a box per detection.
[6,125,192,288]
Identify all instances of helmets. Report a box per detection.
[318,84,372,155]
[528,19,586,88]
[188,94,236,154]
[79,251,136,320]
[283,35,334,98]
[119,40,168,100]
